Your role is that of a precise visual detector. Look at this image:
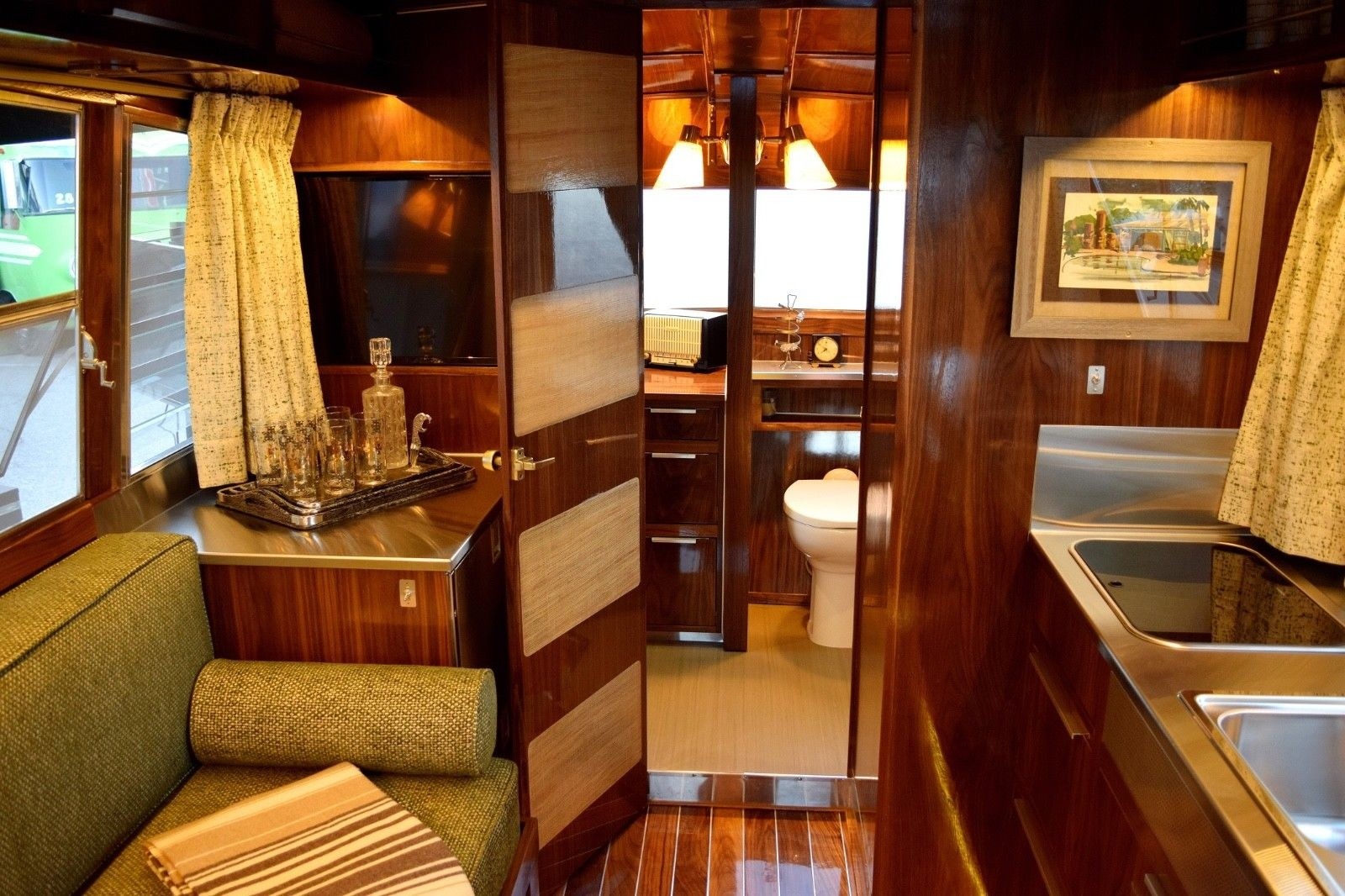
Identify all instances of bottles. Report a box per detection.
[362,338,409,469]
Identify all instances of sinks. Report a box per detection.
[1179,688,1343,896]
[1069,535,1345,654]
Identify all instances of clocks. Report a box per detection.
[813,334,842,363]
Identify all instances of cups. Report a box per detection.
[281,411,320,480]
[350,412,389,485]
[317,407,353,476]
[250,419,290,485]
[278,427,317,500]
[316,419,355,496]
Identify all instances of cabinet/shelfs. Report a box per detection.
[645,365,726,644]
[198,514,510,757]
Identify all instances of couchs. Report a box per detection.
[0,533,541,896]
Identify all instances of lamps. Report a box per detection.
[651,125,836,190]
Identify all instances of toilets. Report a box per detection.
[781,478,861,649]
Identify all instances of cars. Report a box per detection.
[0,145,189,356]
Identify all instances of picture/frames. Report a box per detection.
[1010,137,1272,342]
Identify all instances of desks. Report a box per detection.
[752,360,898,432]
